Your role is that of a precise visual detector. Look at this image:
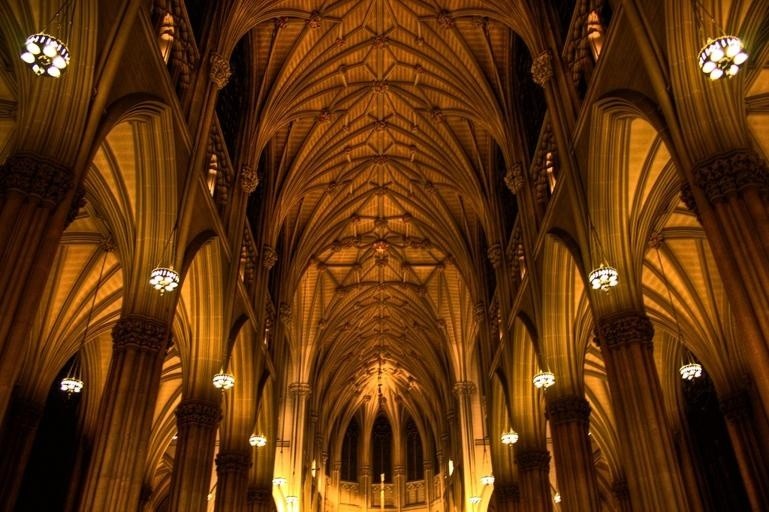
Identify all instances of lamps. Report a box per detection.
[149,219,181,298]
[20,0,77,77]
[501,423,520,448]
[532,347,557,392]
[692,0,750,81]
[58,249,108,401]
[584,213,618,294]
[248,432,268,448]
[656,245,702,381]
[211,354,235,390]
